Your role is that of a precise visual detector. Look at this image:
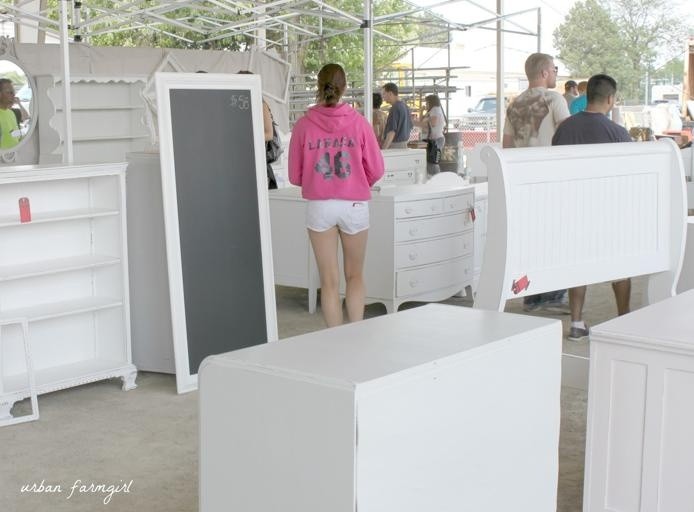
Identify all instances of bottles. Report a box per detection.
[18,197,31,222]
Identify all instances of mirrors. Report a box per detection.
[1,54,39,156]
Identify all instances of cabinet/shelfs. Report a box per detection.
[268,185,477,314]
[196,302,564,512]
[583,287,694,512]
[34,72,155,165]
[0,161,139,427]
[375,149,427,185]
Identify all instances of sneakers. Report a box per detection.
[522,300,573,314]
[567,324,589,341]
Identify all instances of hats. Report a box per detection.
[565,80,577,90]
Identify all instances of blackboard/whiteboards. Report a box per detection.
[158,71,279,394]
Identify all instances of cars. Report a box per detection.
[456,95,510,131]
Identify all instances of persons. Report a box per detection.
[570,81,588,114]
[10,96,29,126]
[552,73,635,341]
[414,95,447,174]
[0,79,21,151]
[373,90,384,144]
[562,81,577,106]
[237,71,283,189]
[500,53,571,309]
[289,64,384,327]
[383,82,414,147]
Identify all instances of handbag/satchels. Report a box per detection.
[265,102,285,164]
[422,138,441,165]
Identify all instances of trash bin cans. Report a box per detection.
[439,133,460,178]
